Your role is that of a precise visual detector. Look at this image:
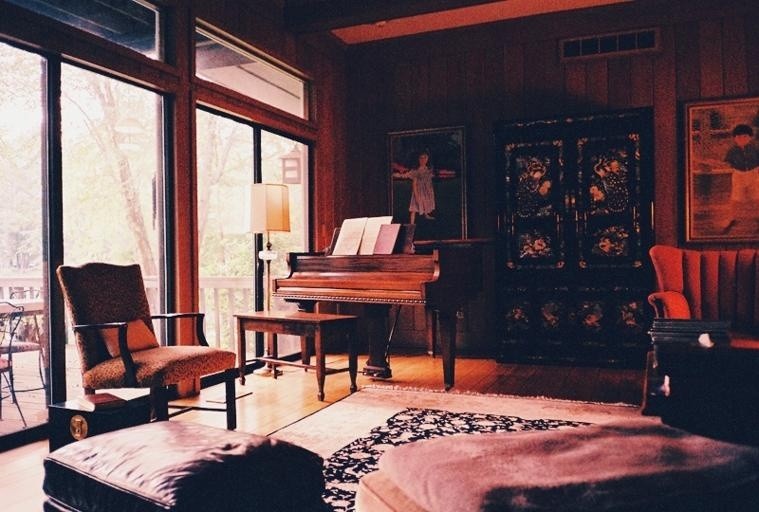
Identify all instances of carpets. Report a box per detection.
[264,384,640,465]
[322,408,594,512]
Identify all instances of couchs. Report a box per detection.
[648,246,759,351]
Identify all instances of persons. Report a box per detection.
[392,151,455,223]
[718,124,759,231]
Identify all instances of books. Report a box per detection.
[332,216,393,255]
[373,223,414,255]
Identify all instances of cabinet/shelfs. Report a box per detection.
[496,279,654,370]
[495,104,657,290]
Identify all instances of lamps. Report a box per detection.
[251,182,291,378]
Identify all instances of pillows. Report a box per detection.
[99,319,159,357]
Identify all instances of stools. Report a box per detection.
[353,415,759,512]
[233,308,360,401]
[43,421,328,511]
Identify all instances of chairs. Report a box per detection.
[1,289,46,390]
[57,263,239,432]
[0,301,28,427]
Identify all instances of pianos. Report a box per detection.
[272,224,495,391]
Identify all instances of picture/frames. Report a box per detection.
[386,124,469,246]
[678,93,759,250]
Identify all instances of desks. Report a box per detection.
[1,299,49,409]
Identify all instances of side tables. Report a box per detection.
[643,316,730,428]
[48,389,152,455]
